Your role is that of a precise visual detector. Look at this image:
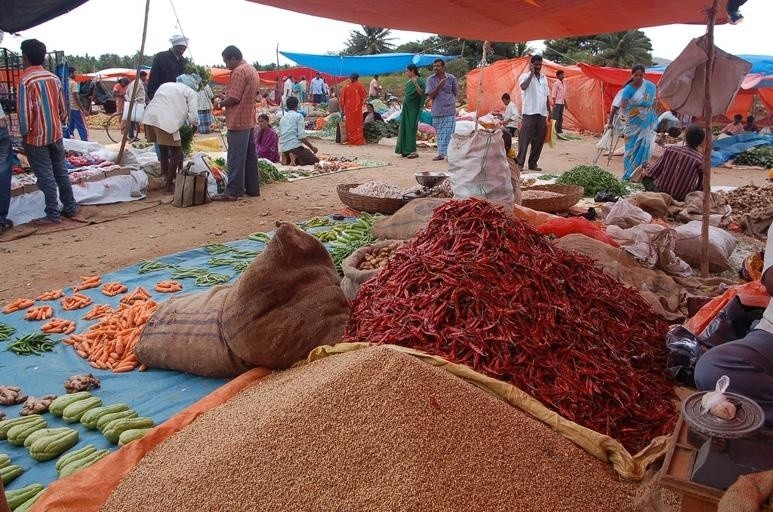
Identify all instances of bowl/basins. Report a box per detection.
[414,172,450,185]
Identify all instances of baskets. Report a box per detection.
[337,181,409,214]
[521,183,586,211]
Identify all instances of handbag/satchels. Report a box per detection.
[123,99,145,124]
[172,162,213,207]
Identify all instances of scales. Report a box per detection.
[402,171,450,205]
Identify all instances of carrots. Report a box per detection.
[3,274,183,373]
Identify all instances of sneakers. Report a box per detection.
[519,165,524,171]
[64,204,80,216]
[1,219,14,231]
[529,167,542,171]
[406,152,419,158]
[211,193,238,201]
[432,155,444,160]
[244,191,260,197]
[48,214,62,223]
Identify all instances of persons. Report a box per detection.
[0,39,79,233]
[142,34,320,201]
[608,65,759,203]
[310,73,340,114]
[66,68,148,140]
[691,222,772,431]
[338,58,459,160]
[491,55,568,205]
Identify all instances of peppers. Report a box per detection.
[342,195,681,456]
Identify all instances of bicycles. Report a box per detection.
[103,90,142,148]
[380,90,402,111]
